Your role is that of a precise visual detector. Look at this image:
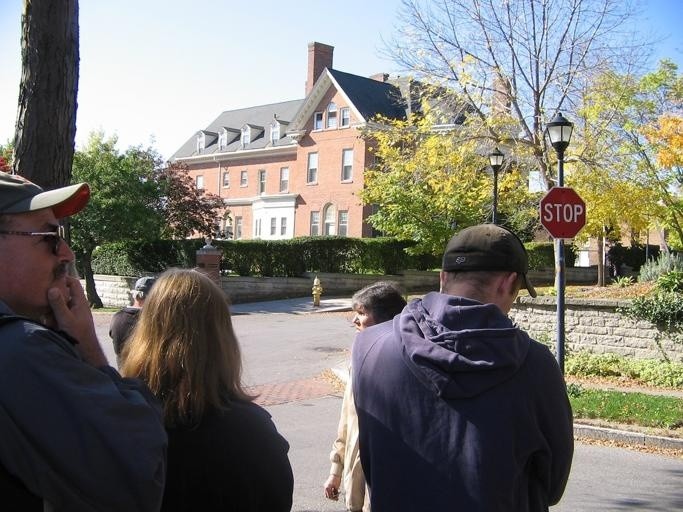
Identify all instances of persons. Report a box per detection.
[348,222,575,511]
[105,275,157,369]
[0,168,170,511]
[321,279,406,511]
[121,265,295,511]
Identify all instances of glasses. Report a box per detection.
[1,225,65,256]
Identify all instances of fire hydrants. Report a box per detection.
[309,276,325,309]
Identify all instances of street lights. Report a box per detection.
[545,112,574,385]
[484,146,503,226]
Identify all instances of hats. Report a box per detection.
[134,276,155,291]
[0,171,91,220]
[441,223,539,298]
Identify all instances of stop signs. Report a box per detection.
[535,186,591,241]
